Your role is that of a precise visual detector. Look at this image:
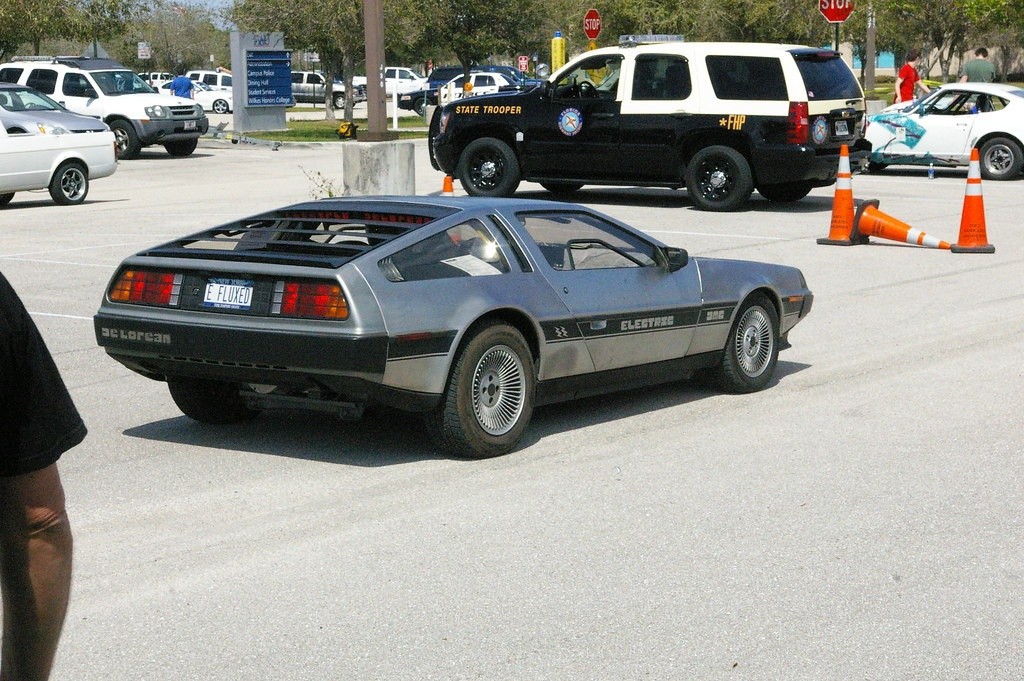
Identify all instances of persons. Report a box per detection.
[958,47,997,83]
[0,270,90,681]
[169,67,194,100]
[893,48,931,104]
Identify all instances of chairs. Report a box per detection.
[460,238,503,272]
[976,95,990,112]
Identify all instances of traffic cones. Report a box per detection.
[947,150,996,255]
[442,176,455,198]
[848,199,951,251]
[816,144,870,247]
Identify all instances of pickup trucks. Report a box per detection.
[352,66,429,96]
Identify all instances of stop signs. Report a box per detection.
[819,1,857,24]
[583,9,603,39]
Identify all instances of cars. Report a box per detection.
[0,83,119,206]
[398,71,541,116]
[91,196,815,460]
[137,68,232,115]
[865,81,1023,182]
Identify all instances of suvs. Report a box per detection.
[290,71,364,110]
[0,55,209,160]
[429,41,873,214]
[427,64,544,89]
